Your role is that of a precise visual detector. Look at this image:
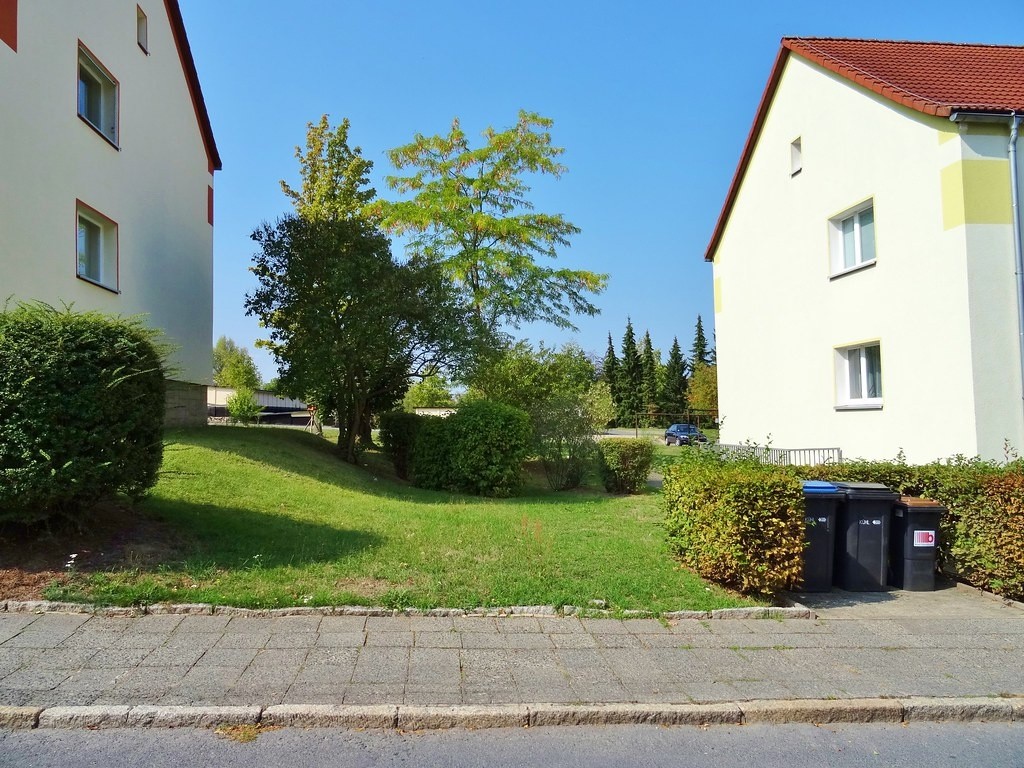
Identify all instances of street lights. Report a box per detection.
[949,111,1024,403]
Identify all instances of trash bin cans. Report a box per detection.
[889,497,949,592]
[786,481,847,593]
[834,482,901,592]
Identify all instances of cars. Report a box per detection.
[664,423,706,446]
